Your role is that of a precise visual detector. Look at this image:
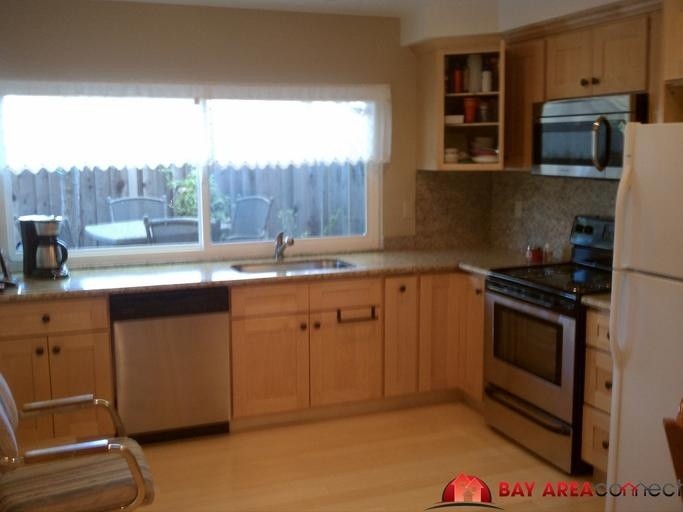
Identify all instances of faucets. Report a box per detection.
[273,231,295,263]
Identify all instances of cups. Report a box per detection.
[450,68,463,93]
[443,146,465,164]
[481,69,492,93]
[462,96,477,123]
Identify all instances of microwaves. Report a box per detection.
[487,261,611,303]
[531,93,640,179]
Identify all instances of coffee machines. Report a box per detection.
[17,214,69,281]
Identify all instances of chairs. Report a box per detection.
[1,375,155,512]
[104,195,168,223]
[142,215,220,244]
[219,195,273,241]
[58,216,75,248]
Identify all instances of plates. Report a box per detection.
[471,155,498,163]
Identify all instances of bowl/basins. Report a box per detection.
[443,115,463,124]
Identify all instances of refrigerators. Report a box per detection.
[591,118,683,512]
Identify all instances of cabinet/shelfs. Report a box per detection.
[384,270,458,403]
[454,272,487,419]
[0,298,116,440]
[227,275,384,432]
[503,30,546,173]
[415,32,506,173]
[547,2,651,99]
[580,308,613,473]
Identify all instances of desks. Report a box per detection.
[84,218,229,246]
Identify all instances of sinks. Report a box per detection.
[228,257,360,277]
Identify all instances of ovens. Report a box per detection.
[476,280,581,477]
